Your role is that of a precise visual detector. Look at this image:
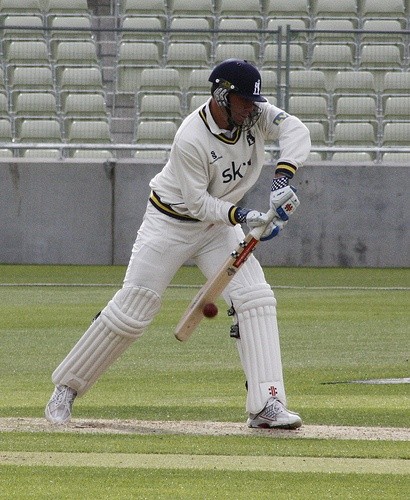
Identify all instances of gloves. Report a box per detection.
[237,207,284,241]
[270,176,300,221]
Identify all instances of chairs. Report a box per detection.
[0,0,410,162]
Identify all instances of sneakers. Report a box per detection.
[45,384,78,426]
[246,397,303,430]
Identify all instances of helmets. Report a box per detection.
[208,59,267,132]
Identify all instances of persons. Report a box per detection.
[45,60,312,429]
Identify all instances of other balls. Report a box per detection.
[203,303,218,318]
